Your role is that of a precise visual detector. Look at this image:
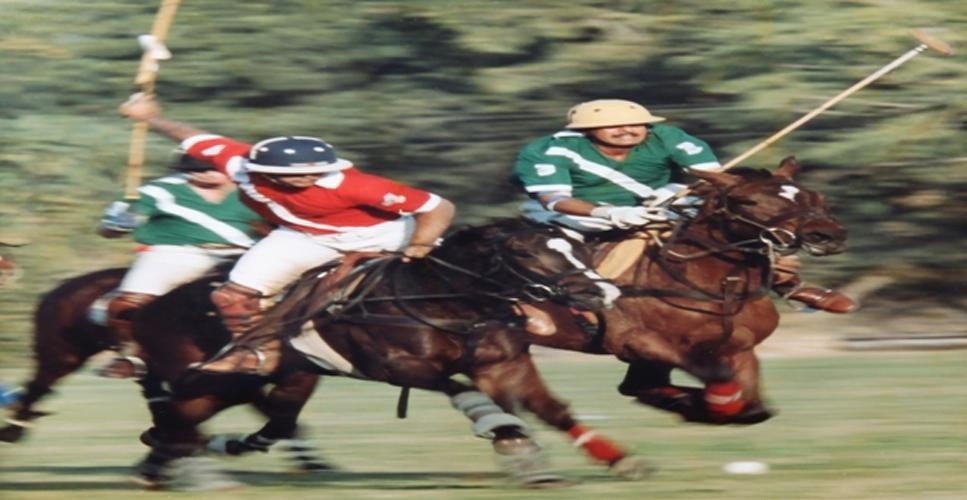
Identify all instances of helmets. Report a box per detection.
[565,100,666,129]
[169,154,217,172]
[244,137,353,174]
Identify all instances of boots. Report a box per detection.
[783,278,856,313]
[513,300,557,336]
[99,317,158,380]
[185,310,282,376]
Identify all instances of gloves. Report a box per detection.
[102,202,148,231]
[596,207,670,229]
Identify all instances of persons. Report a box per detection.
[94,149,280,380]
[118,91,558,378]
[513,99,856,314]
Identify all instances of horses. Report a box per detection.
[460,152,848,480]
[0,264,327,490]
[129,215,624,493]
